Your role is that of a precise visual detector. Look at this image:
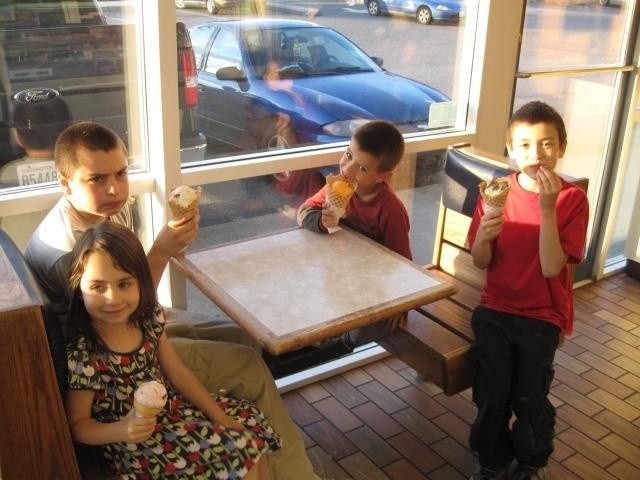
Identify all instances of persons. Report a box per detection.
[296,121,412,345]
[63,222,282,479]
[264,57,310,96]
[256,116,326,207]
[23,120,326,480]
[2,85,81,186]
[465,100,590,479]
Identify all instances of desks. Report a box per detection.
[171,221,457,357]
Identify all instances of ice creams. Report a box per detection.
[326,175,360,234]
[134,381,169,419]
[478,177,512,215]
[168,185,201,216]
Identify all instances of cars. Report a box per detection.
[177,0,264,16]
[189,21,456,191]
[364,1,463,25]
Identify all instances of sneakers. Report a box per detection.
[469,466,508,480]
[509,459,547,480]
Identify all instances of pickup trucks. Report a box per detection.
[0,0,208,169]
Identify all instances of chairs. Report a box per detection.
[0,228,258,478]
[366,145,589,397]
